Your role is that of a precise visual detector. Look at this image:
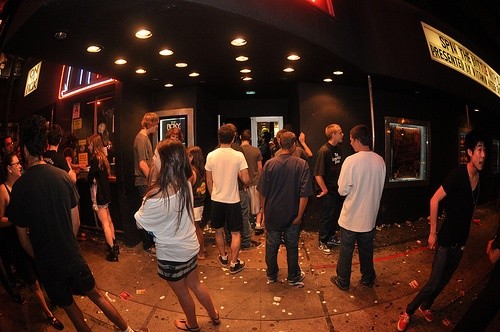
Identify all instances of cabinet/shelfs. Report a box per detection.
[76,174,126,234]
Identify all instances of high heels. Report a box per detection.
[107,245,119,262]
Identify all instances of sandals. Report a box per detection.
[174,319,201,332]
[209,306,220,326]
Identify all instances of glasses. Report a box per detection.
[9,162,21,166]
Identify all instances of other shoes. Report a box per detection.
[242,240,262,250]
[46,312,64,330]
[255,228,264,236]
[330,274,350,291]
[358,277,374,288]
[197,254,205,260]
[143,247,157,257]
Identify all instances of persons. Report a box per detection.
[313,124,344,255]
[330,125,387,290]
[0,113,312,332]
[450,228,500,332]
[388,129,487,332]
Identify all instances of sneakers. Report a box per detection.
[418,305,434,323]
[266,272,279,283]
[229,258,245,274]
[397,309,411,331]
[217,252,229,267]
[288,272,306,286]
[318,240,332,255]
[327,235,341,246]
